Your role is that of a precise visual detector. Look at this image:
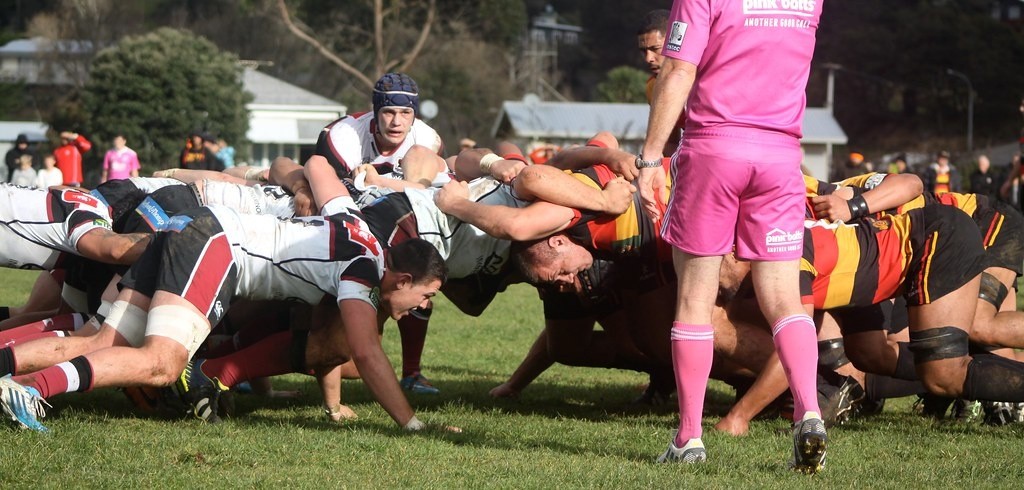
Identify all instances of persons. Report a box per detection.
[0,163,319,330]
[2,156,467,437]
[170,148,638,424]
[639,1,830,474]
[180,132,236,175]
[53,131,92,190]
[36,154,64,192]
[6,134,41,180]
[312,71,453,400]
[100,136,141,185]
[14,156,39,190]
[446,113,1023,435]
[0,182,155,272]
[0,168,362,422]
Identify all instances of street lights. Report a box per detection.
[822,62,843,178]
[946,68,974,152]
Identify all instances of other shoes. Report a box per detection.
[629,389,673,407]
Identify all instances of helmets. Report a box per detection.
[373,73,418,135]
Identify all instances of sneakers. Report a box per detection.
[787,412,827,473]
[1,373,49,432]
[400,372,439,393]
[654,437,706,463]
[754,376,1024,427]
[122,357,234,424]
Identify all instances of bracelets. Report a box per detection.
[327,404,342,417]
[402,416,425,433]
[636,155,664,170]
